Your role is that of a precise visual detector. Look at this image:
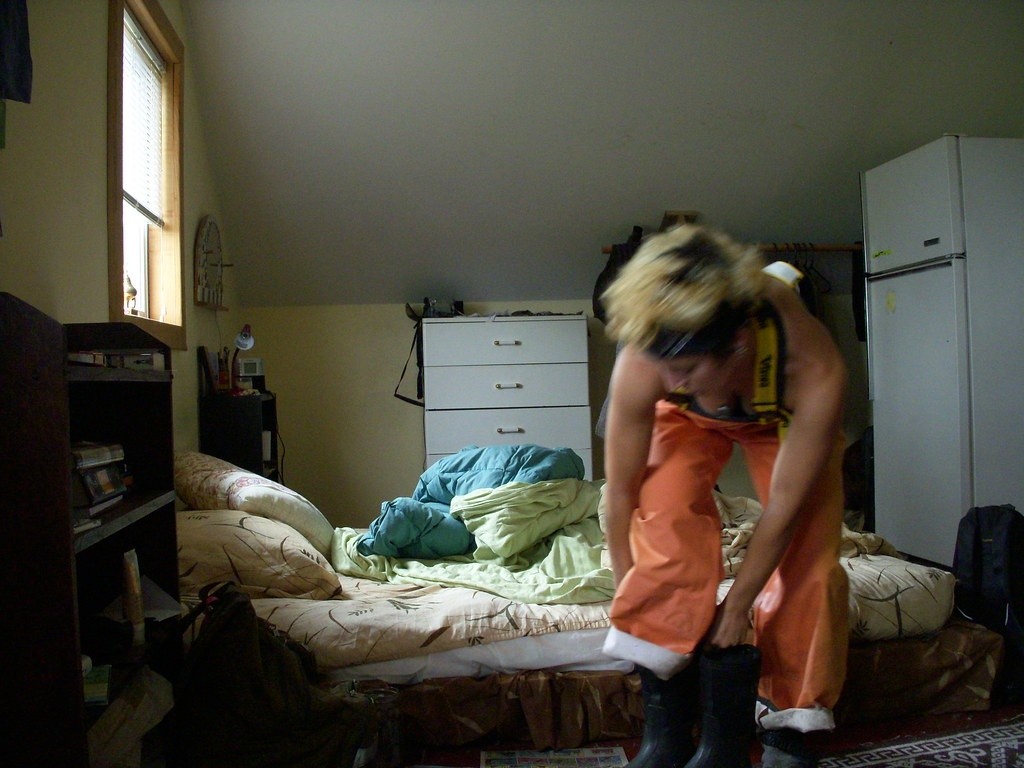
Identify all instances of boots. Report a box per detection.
[621,662,698,768]
[681,643,763,768]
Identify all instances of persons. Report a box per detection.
[598,224,850,768]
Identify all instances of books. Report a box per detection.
[73,445,127,534]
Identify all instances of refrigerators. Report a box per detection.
[859,133,1024,566]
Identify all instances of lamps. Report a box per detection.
[227,324,254,398]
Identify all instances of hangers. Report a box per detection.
[769,239,831,295]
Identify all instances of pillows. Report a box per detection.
[177,506,352,602]
[175,448,337,562]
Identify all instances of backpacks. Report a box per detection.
[951,502,1024,708]
[152,584,367,768]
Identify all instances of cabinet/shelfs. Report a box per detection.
[0,290,187,768]
[423,314,594,485]
[198,391,277,483]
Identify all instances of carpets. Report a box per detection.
[813,702,1024,768]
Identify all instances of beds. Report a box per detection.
[175,525,1009,752]
